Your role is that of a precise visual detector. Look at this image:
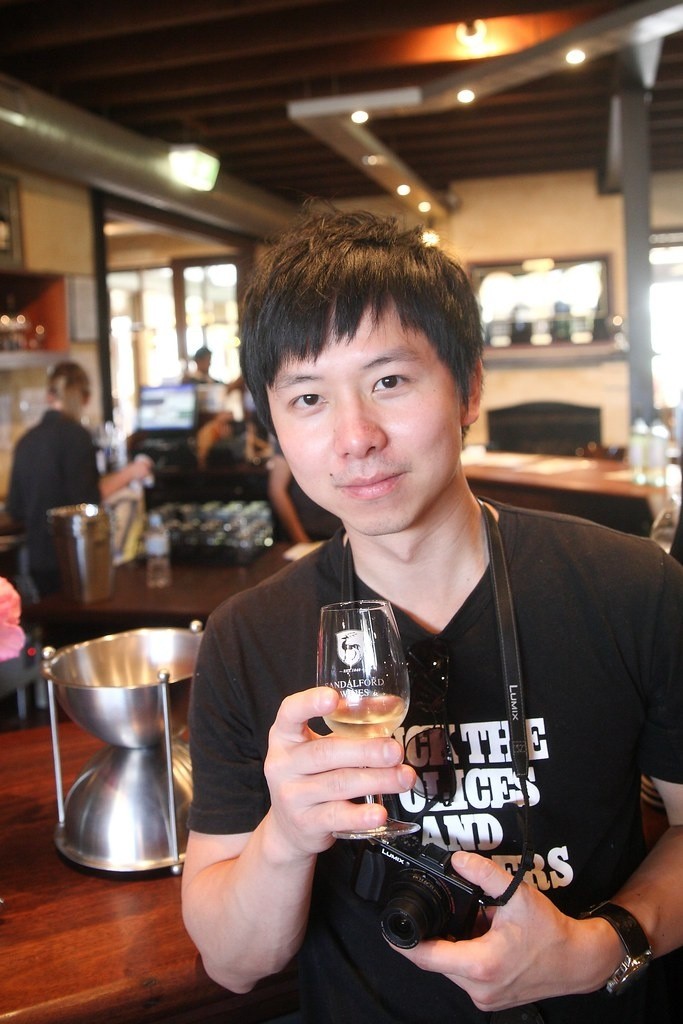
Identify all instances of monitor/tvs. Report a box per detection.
[138,384,199,433]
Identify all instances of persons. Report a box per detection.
[5,359,157,595]
[180,213,682,1023]
[181,341,342,546]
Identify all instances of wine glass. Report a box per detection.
[316,600,421,839]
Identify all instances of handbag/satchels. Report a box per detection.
[206,423,274,467]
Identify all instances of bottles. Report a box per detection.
[649,409,670,487]
[553,303,570,343]
[629,410,649,485]
[650,465,682,555]
[512,306,532,346]
[143,516,171,588]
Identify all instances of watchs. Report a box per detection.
[584,902,653,996]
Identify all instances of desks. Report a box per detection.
[0,722,210,1024]
[462,449,672,538]
[29,543,324,647]
